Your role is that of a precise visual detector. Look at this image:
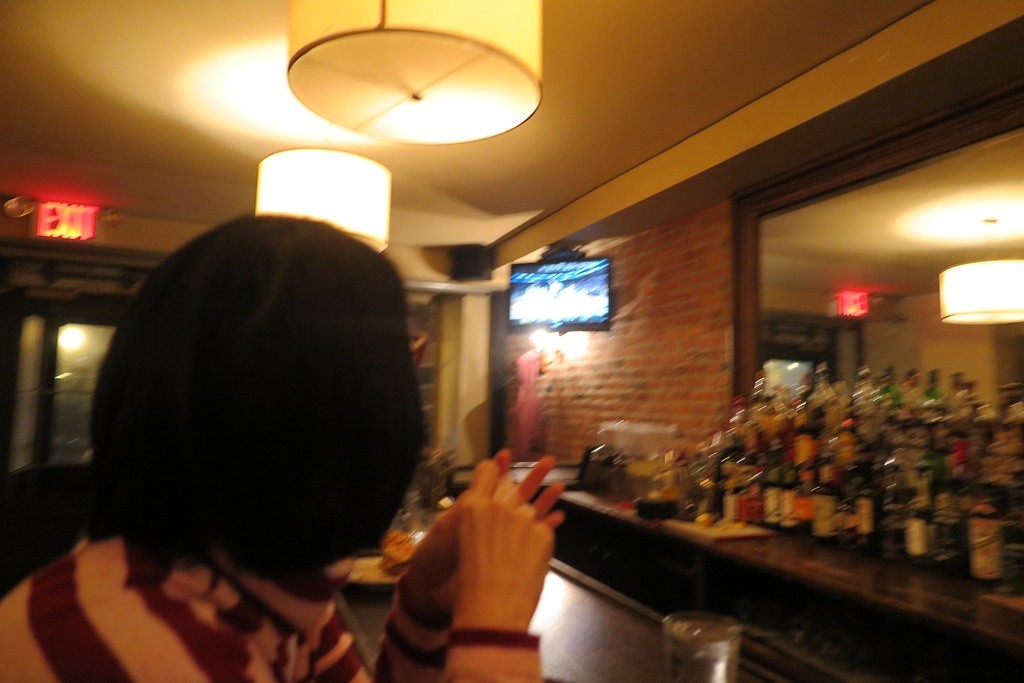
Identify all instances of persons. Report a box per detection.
[0,214,566,683]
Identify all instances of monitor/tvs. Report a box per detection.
[508,256,614,332]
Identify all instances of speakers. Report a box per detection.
[450,245,492,281]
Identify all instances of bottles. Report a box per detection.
[685,361,1024,580]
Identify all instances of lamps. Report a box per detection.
[255,149,393,252]
[937,218,1024,325]
[287,0,545,144]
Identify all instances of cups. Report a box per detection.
[663,616,741,683]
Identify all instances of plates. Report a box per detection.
[673,520,773,541]
[348,557,397,585]
